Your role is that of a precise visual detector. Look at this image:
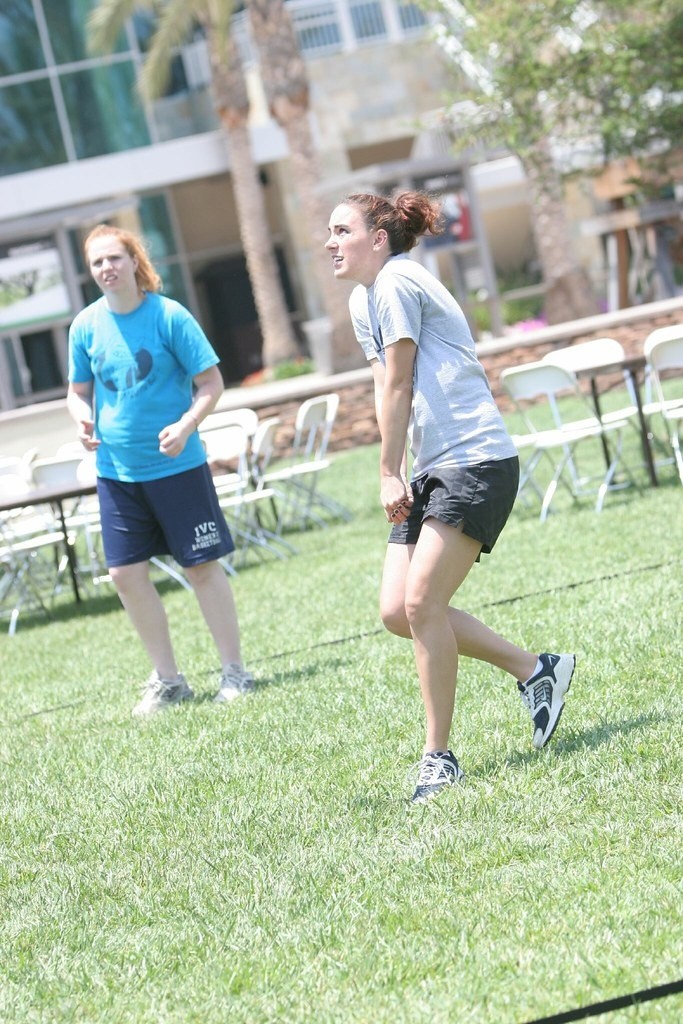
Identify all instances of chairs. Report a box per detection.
[0,395,341,635]
[500,322,683,520]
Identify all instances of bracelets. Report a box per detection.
[188,414,198,431]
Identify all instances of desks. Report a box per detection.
[574,357,663,489]
[0,479,99,602]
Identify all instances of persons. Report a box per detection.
[325,190,576,804]
[66,225,254,716]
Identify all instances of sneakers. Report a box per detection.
[215,664,252,702]
[517,653,576,750]
[132,671,191,715]
[407,750,464,803]
